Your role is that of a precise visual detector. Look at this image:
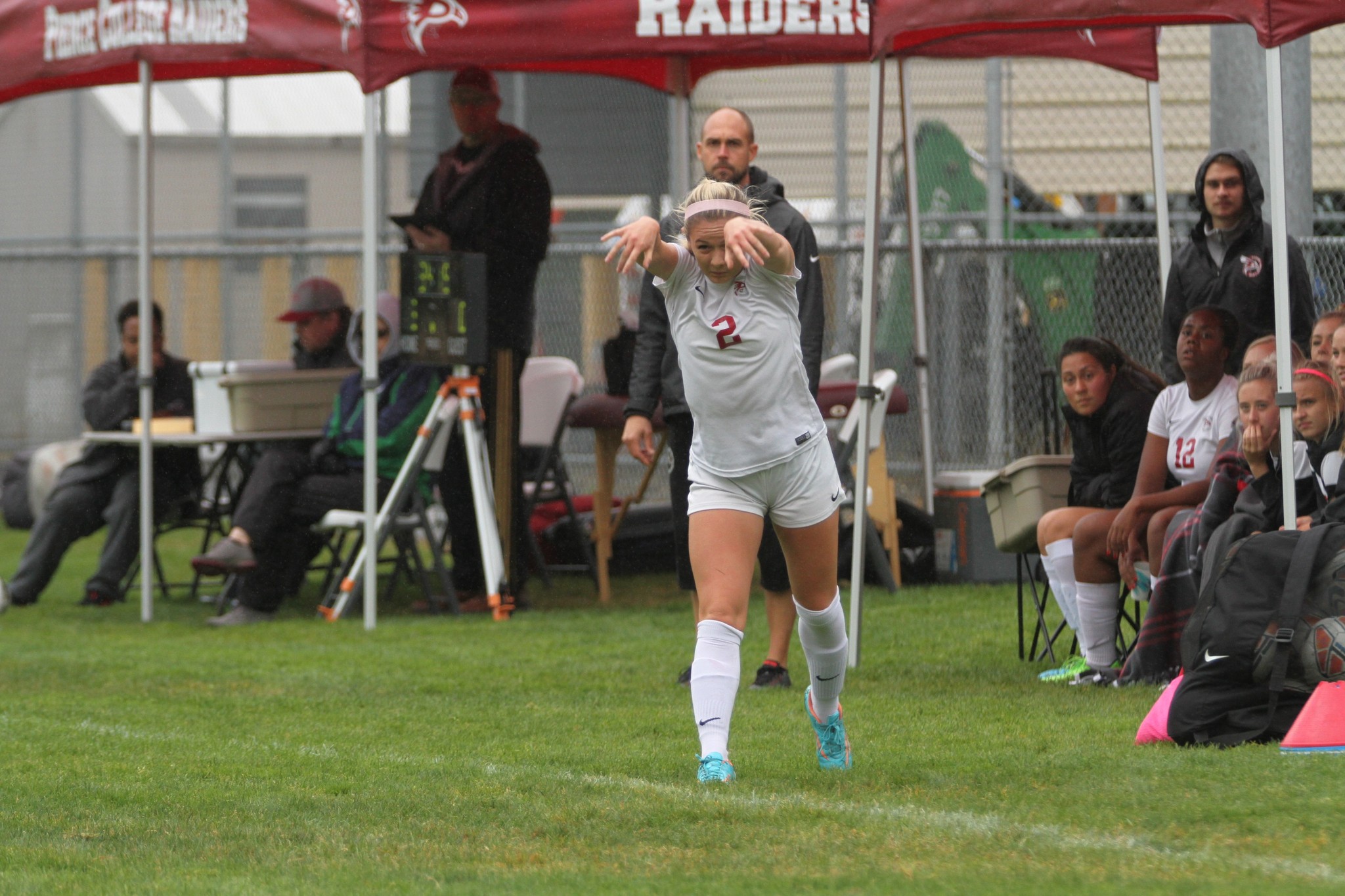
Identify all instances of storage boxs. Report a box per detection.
[977,454,1075,557]
[188,359,364,437]
[930,469,1039,585]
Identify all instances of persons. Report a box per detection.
[403,66,552,614]
[189,277,439,626]
[622,105,824,688]
[0,300,203,610]
[1038,149,1345,744]
[599,179,854,783]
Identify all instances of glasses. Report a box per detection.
[359,328,389,340]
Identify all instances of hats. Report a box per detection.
[450,62,499,102]
[277,278,351,322]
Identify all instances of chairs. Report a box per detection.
[817,352,898,596]
[95,354,585,619]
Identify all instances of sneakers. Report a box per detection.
[804,685,852,770]
[1039,651,1124,682]
[696,752,735,785]
[678,665,692,686]
[751,660,791,691]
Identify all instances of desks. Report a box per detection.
[80,431,326,603]
[567,381,912,609]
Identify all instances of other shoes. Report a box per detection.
[453,592,489,614]
[78,587,119,606]
[205,605,272,628]
[192,539,257,576]
[411,589,469,612]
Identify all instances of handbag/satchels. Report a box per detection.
[1136,667,1185,746]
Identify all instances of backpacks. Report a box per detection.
[1167,521,1345,745]
